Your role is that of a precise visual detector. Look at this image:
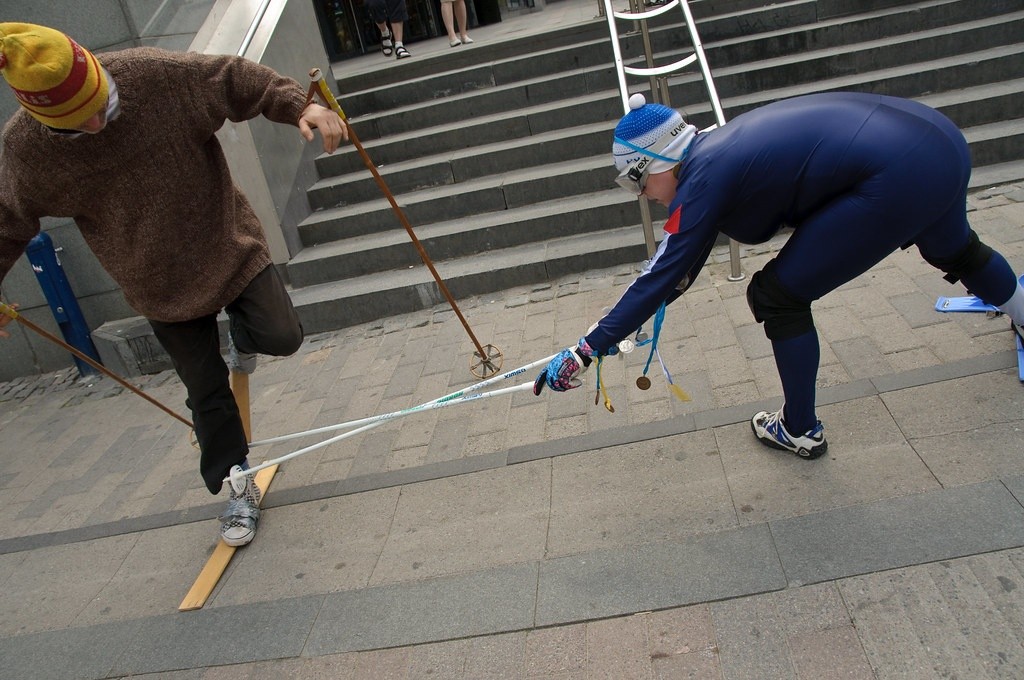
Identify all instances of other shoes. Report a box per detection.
[460,37,474,44]
[447,37,461,47]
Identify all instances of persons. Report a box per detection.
[533,92,1024,463]
[0,21,352,545]
[441,0,474,47]
[364,0,411,59]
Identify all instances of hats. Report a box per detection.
[0,21,110,130]
[611,92,697,174]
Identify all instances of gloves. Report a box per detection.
[532,335,597,396]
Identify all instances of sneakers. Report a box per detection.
[220,458,261,547]
[1011,318,1024,349]
[228,329,257,374]
[751,402,829,460]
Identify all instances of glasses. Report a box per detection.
[613,168,643,197]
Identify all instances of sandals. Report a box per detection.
[379,28,394,57]
[394,46,413,59]
